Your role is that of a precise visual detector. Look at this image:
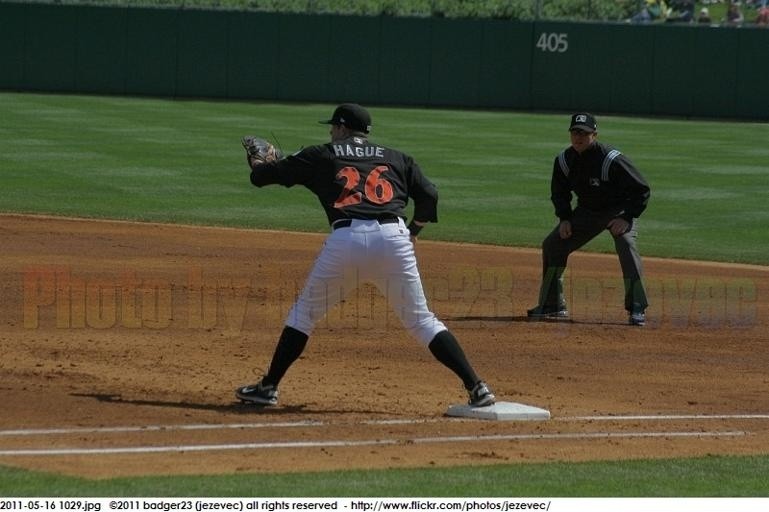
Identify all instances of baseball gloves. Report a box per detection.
[239,132,283,168]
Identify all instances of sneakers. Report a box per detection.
[628,310,646,327]
[236,379,278,406]
[468,381,494,406]
[526,304,569,318]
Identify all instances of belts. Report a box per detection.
[334,217,399,229]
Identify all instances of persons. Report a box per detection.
[621,0,769,29]
[526,112,652,327]
[233,101,499,410]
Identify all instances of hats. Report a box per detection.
[570,113,597,133]
[319,104,371,134]
